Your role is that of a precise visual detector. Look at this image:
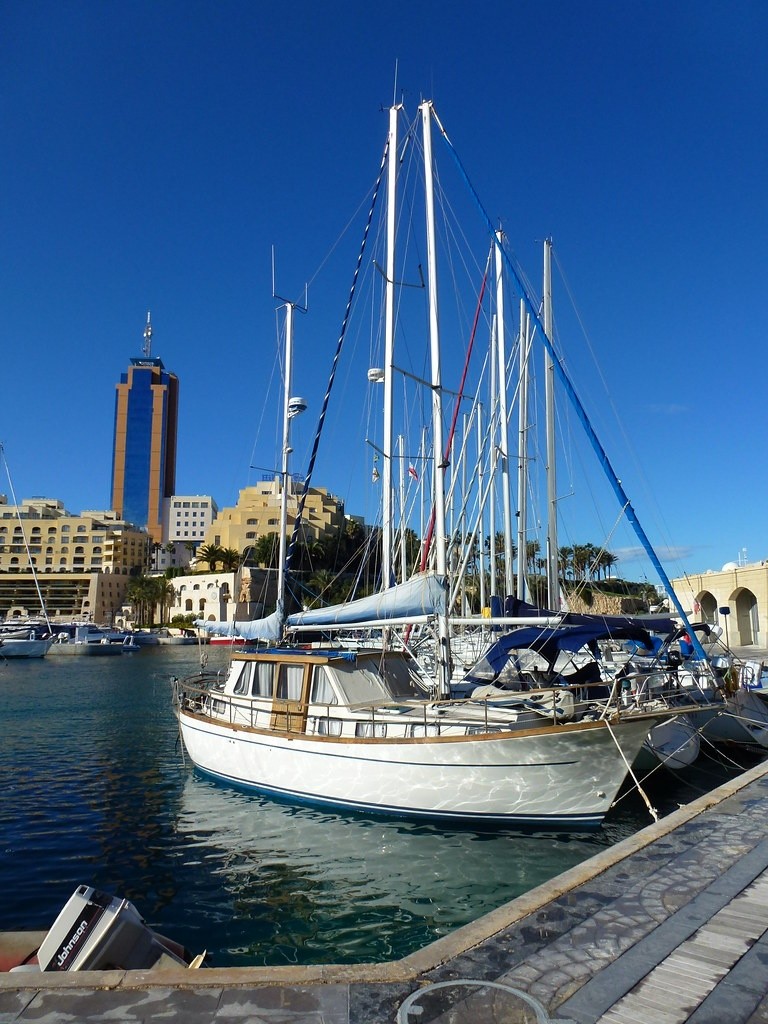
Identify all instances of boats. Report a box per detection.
[46,638,124,656]
[69,625,258,646]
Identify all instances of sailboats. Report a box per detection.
[0,443,56,659]
[170,58,768,828]
